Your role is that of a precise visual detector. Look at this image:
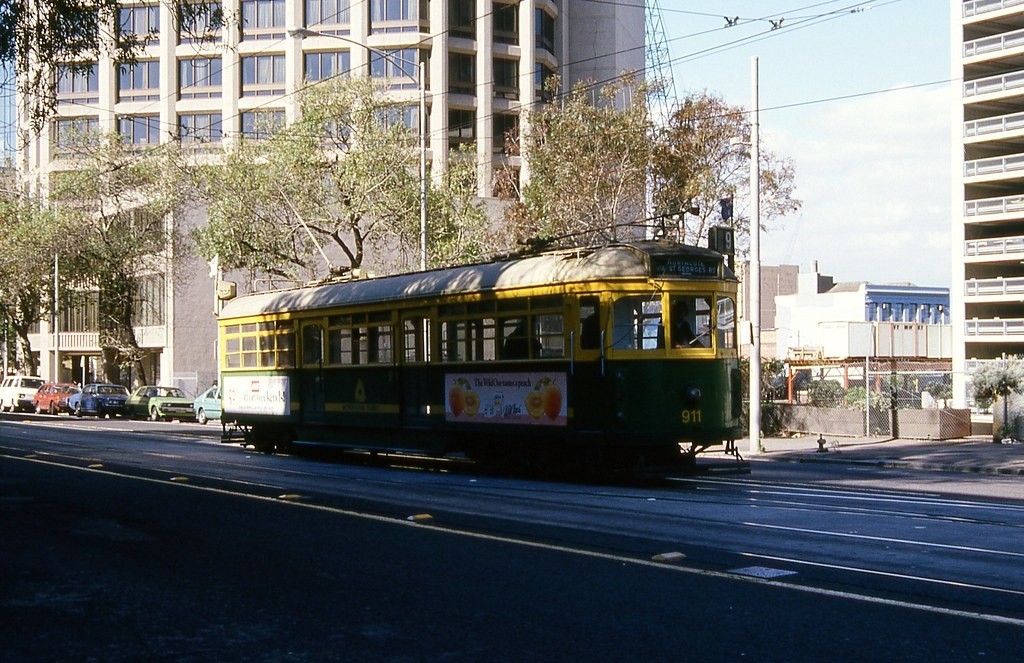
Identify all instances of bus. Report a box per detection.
[211,179,754,479]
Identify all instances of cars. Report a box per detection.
[192,385,222,425]
[66,383,130,419]
[32,383,82,415]
[124,385,196,422]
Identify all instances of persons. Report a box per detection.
[658,302,705,348]
[212,380,218,388]
[504,319,541,360]
[581,303,602,349]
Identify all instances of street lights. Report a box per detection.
[288,24,426,271]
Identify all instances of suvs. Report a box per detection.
[0,376,50,412]
